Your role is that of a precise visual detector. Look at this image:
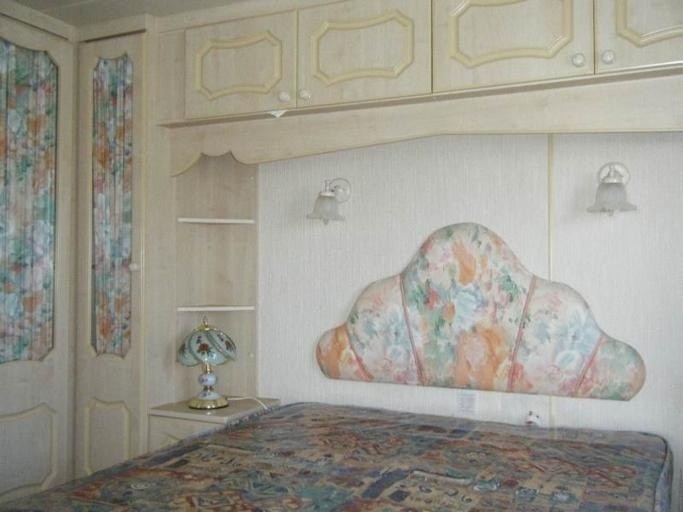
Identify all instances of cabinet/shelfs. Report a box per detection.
[431,0,683,102]
[174,215,256,313]
[182,1,431,120]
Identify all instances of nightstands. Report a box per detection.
[148,396,280,452]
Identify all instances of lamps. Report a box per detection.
[303,179,350,226]
[585,161,639,218]
[177,315,236,411]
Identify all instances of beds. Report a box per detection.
[0,401,670,511]
